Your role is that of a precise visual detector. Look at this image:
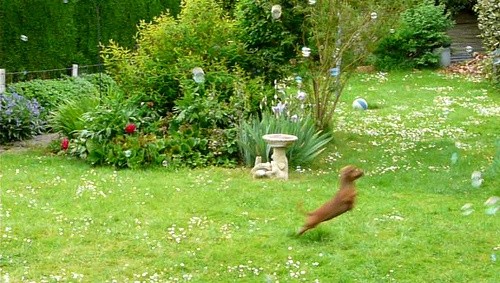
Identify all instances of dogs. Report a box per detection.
[293,164,365,240]
